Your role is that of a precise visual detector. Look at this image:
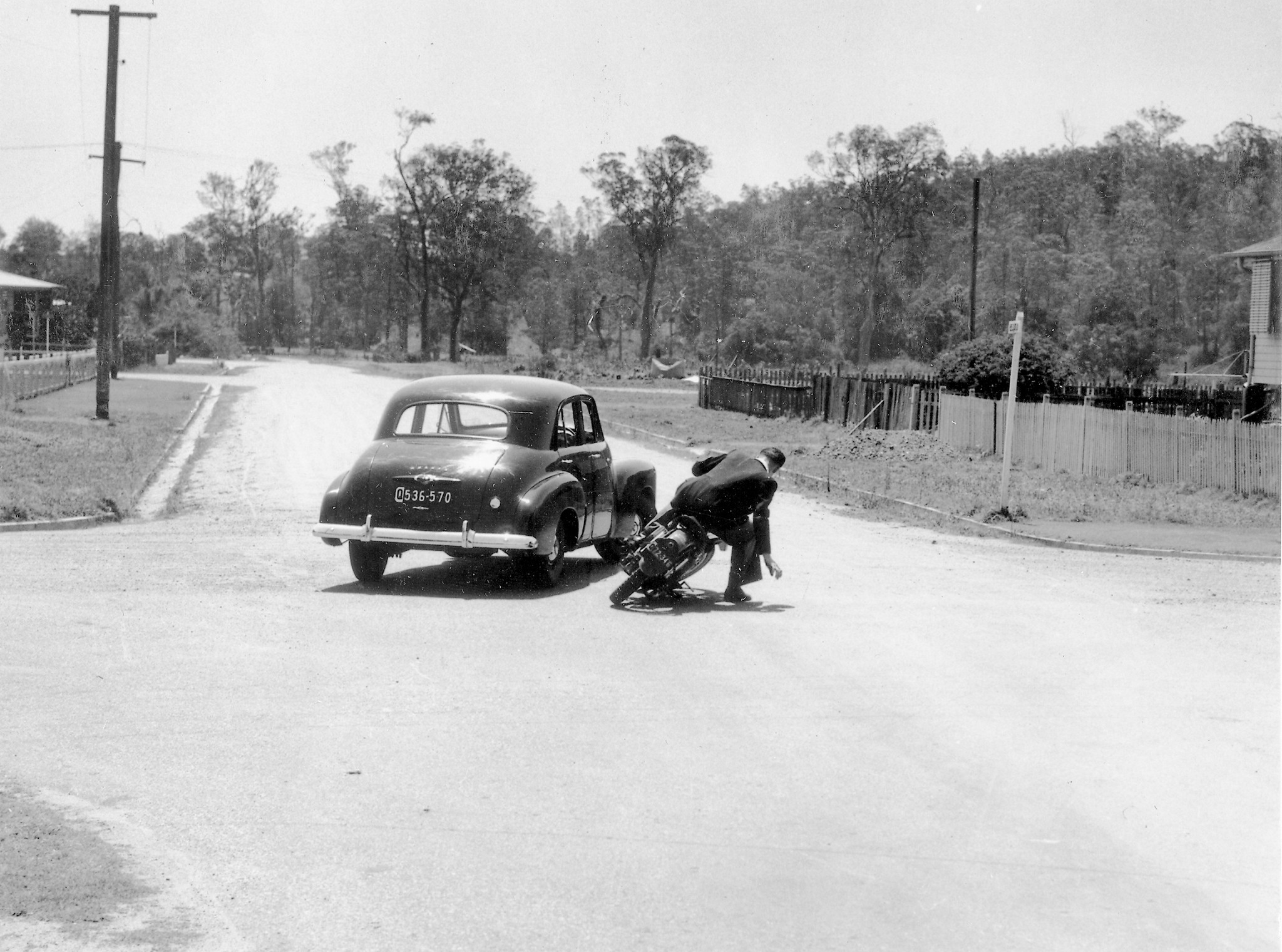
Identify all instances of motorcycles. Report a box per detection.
[609,447,729,605]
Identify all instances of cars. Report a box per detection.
[312,374,658,589]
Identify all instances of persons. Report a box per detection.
[640,446,785,601]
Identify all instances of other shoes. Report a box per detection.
[723,589,751,601]
[625,534,643,548]
